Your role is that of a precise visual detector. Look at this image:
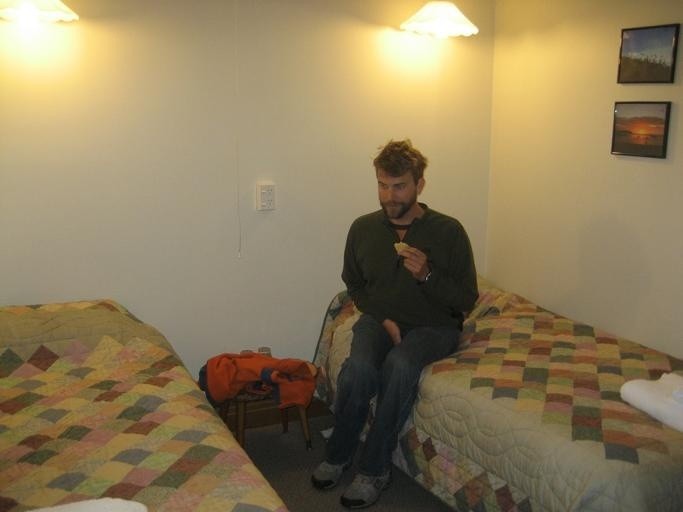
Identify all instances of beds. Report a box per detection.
[0,300,288,512]
[311,273,682,511]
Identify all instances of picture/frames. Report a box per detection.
[610,22,681,159]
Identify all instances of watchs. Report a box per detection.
[425,271,432,280]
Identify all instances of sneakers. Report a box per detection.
[341,463,392,509]
[311,456,352,489]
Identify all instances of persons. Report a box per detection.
[310,138,479,509]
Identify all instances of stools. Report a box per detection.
[218,389,309,448]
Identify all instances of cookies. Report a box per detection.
[394,242,408,253]
